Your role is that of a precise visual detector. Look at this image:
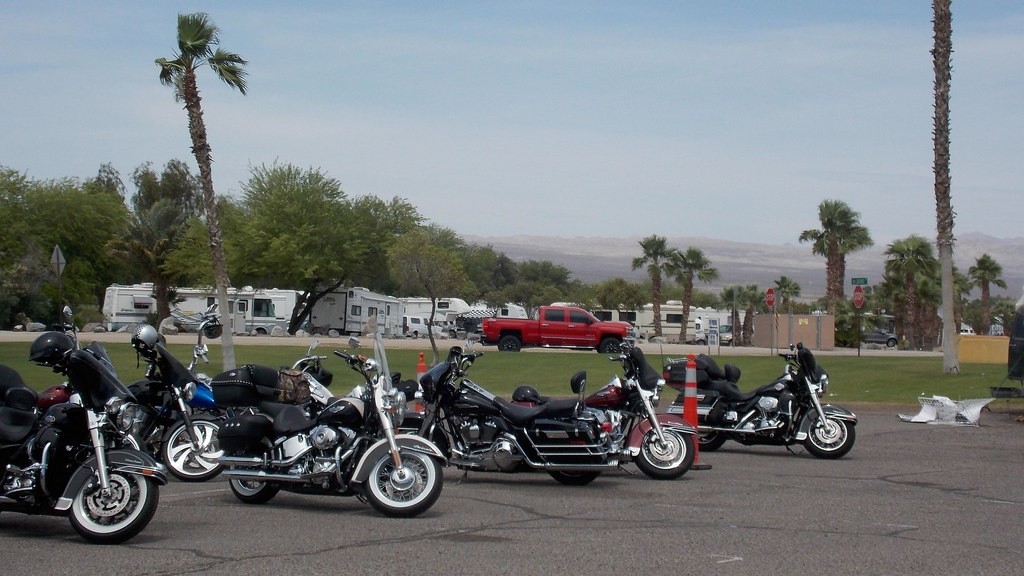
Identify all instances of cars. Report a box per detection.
[862,326,898,348]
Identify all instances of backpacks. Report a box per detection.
[280,370,310,405]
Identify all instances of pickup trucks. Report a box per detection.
[478,305,635,354]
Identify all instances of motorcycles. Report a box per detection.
[0,330,170,545]
[292,338,701,488]
[35,301,230,482]
[658,342,862,460]
[206,334,451,520]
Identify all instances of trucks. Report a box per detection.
[103,280,755,347]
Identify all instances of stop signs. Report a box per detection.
[765,287,776,307]
[853,286,865,309]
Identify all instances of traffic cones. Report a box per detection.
[681,353,715,472]
[413,351,428,416]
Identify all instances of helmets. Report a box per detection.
[29,331,74,361]
[204,324,222,339]
[513,386,538,401]
[303,359,335,389]
[131,324,157,350]
